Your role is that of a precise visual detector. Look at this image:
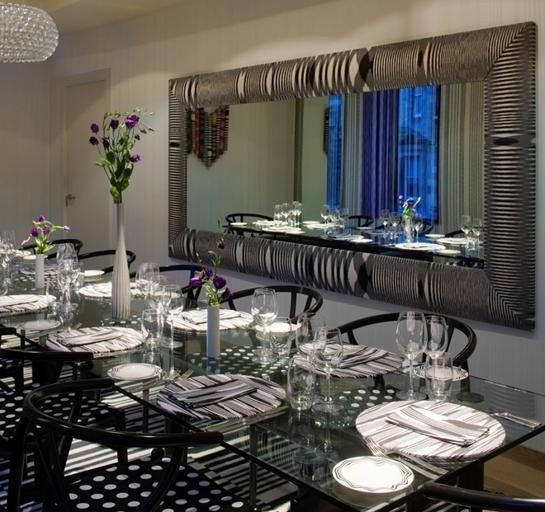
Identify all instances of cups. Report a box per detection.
[411,213,423,242]
[424,351,455,402]
[148,275,172,309]
[460,213,471,237]
[295,311,327,357]
[251,286,280,365]
[471,217,482,245]
[140,307,164,351]
[134,262,162,307]
[423,315,450,358]
[394,309,429,401]
[319,204,330,228]
[390,212,401,240]
[267,317,294,364]
[310,326,346,416]
[159,284,185,382]
[330,209,340,232]
[273,201,303,227]
[380,209,390,235]
[286,354,315,412]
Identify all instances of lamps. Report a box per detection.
[2,3,59,66]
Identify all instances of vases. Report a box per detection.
[188,301,234,358]
[21,254,71,287]
[89,203,157,319]
[402,216,414,242]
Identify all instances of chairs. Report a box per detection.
[73,249,137,276]
[19,238,84,259]
[222,212,275,235]
[376,218,435,237]
[421,482,544,512]
[445,226,484,238]
[327,310,478,376]
[0,322,128,512]
[348,214,375,230]
[218,283,323,344]
[23,377,277,512]
[128,263,210,311]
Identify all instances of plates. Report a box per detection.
[78,269,106,277]
[23,253,48,261]
[413,365,471,382]
[307,223,345,229]
[393,242,447,253]
[437,237,485,245]
[229,222,248,226]
[349,238,373,244]
[331,454,416,495]
[262,225,303,232]
[106,362,163,382]
[253,321,302,333]
[20,319,62,332]
[304,220,321,225]
[424,234,445,238]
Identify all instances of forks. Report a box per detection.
[362,434,442,482]
[371,437,450,475]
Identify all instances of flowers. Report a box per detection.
[20,214,71,256]
[189,232,230,308]
[89,106,154,204]
[397,194,421,217]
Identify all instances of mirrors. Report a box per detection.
[168,21,538,331]
